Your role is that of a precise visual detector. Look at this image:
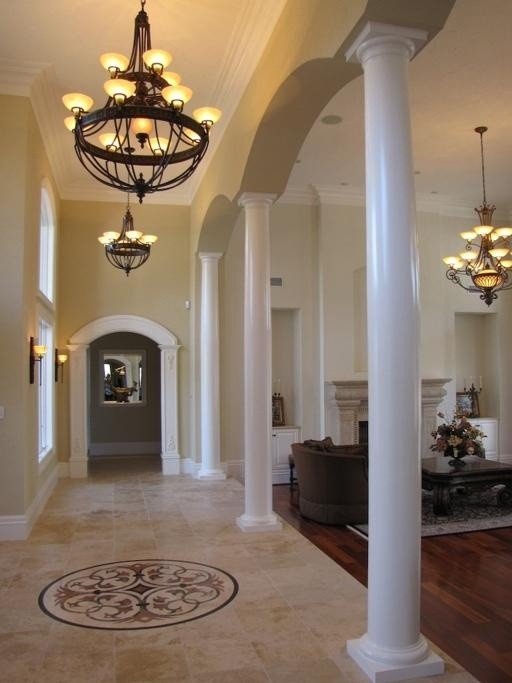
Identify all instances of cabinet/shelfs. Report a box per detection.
[468,419,498,461]
[272,427,301,484]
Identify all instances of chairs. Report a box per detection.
[289,443,368,525]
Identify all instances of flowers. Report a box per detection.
[430,411,485,452]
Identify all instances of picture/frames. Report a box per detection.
[457,392,482,418]
[272,397,285,426]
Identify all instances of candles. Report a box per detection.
[471,376,473,385]
[480,376,482,388]
[464,378,466,387]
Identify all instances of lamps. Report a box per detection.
[62,0,221,202]
[96,164,157,276]
[443,126,512,307]
[55,348,68,384]
[30,337,47,386]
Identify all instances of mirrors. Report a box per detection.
[98,349,148,407]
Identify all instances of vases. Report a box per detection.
[449,446,468,466]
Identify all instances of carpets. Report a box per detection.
[345,486,512,541]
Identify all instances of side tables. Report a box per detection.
[288,455,299,505]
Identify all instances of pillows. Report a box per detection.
[326,443,368,456]
[304,437,333,451]
